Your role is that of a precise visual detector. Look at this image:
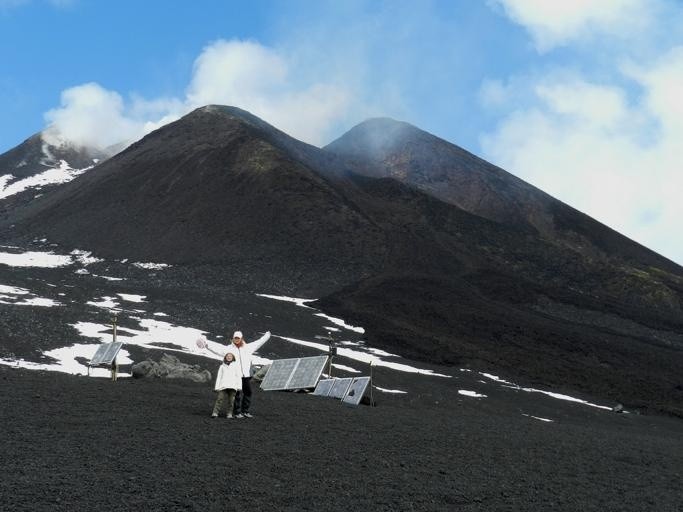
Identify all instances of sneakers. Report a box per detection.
[212,413,252,418]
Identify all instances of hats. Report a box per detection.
[234,331,243,339]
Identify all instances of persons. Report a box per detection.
[205,330,271,419]
[211,353,242,419]
